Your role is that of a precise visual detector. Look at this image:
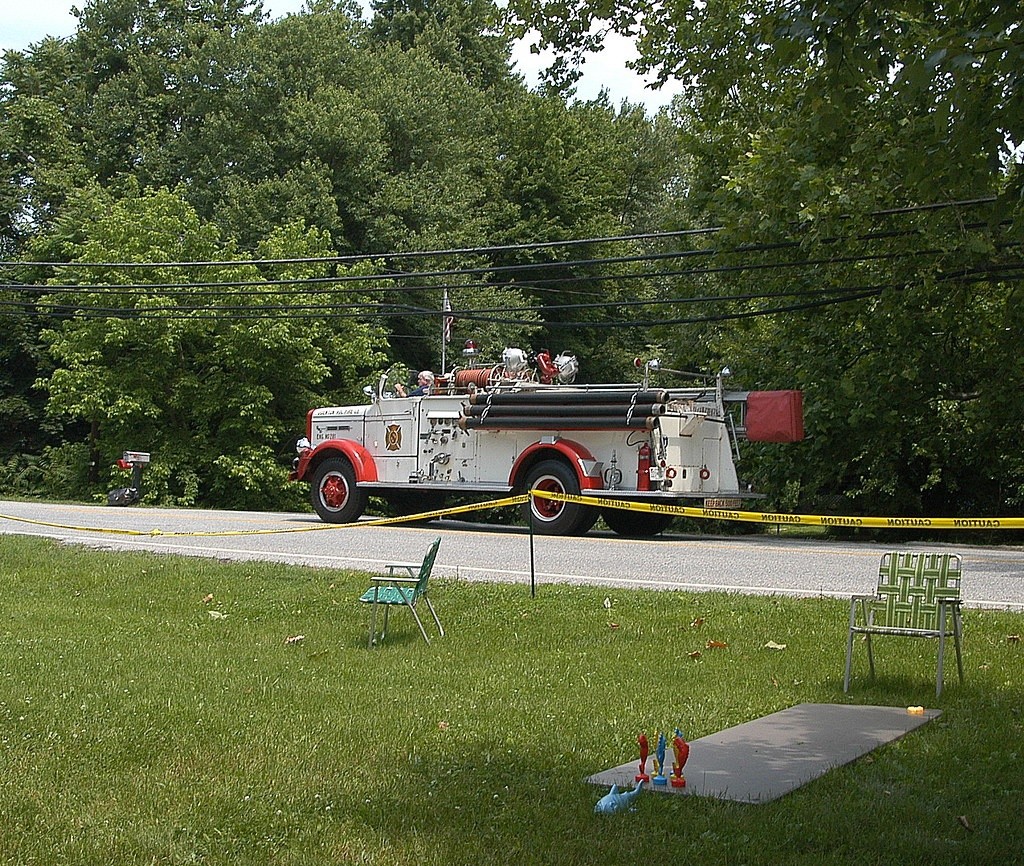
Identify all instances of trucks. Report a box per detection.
[292,339,806,537]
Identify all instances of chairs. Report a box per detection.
[843,553,964,699]
[358,537,444,650]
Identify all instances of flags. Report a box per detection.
[443,289,454,343]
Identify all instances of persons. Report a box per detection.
[394,371,434,398]
